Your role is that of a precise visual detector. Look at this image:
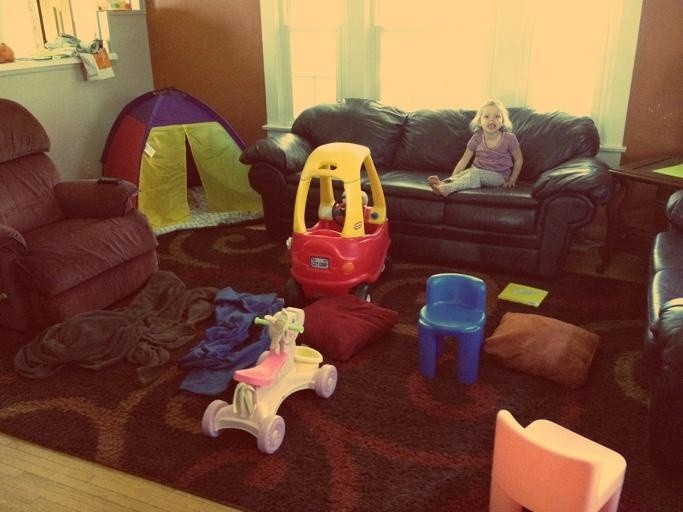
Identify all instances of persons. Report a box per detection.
[425,99,522,202]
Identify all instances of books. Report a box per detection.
[497,282,549,308]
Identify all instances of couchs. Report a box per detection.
[646,191,683,474]
[237,97,614,277]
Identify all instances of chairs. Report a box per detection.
[485,413,628,512]
[0,97,157,351]
[417,274,485,385]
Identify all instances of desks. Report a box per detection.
[608,149,683,269]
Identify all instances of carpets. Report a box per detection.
[4,220,683,512]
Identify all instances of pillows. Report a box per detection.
[482,312,602,388]
[284,291,398,362]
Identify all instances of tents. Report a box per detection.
[98,87,264,235]
